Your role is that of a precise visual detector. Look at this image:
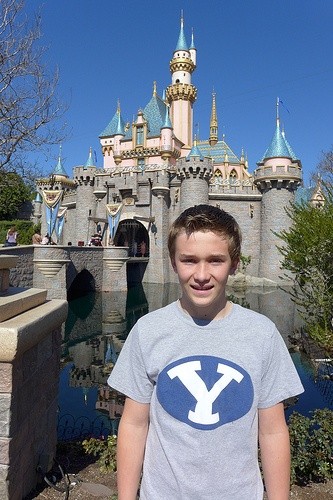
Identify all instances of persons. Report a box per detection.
[107,202,306,500]
[5,227,18,245]
[32,230,103,246]
